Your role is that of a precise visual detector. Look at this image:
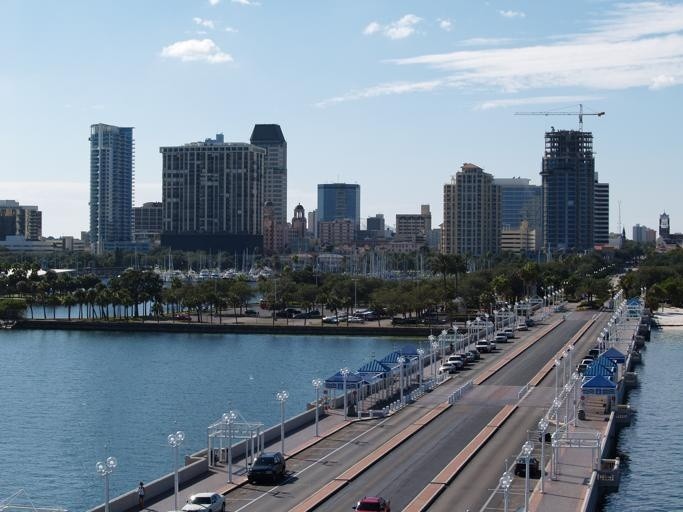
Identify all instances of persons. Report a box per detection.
[137,480,145,508]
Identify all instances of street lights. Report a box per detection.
[220,408,238,484]
[435,286,533,355]
[415,347,426,390]
[310,375,326,438]
[497,471,515,512]
[397,355,408,403]
[166,429,186,512]
[94,455,117,512]
[430,341,440,381]
[520,439,535,512]
[273,388,290,456]
[540,283,635,496]
[339,366,352,421]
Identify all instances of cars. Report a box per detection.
[352,495,391,512]
[446,353,465,371]
[437,362,457,375]
[513,454,540,479]
[457,317,535,365]
[180,491,226,512]
[322,310,365,325]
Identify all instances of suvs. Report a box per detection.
[246,451,286,485]
